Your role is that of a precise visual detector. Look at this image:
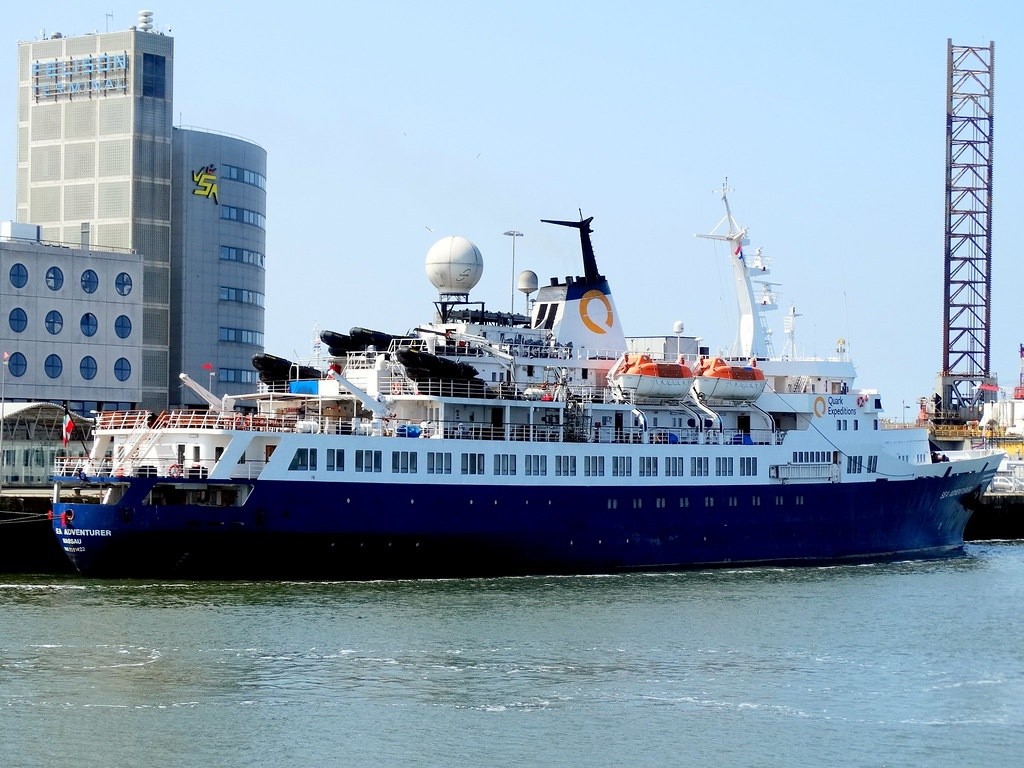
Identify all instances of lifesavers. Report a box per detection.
[234,417,249,429]
[168,464,181,478]
[857,396,865,408]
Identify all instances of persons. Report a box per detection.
[842,383,848,394]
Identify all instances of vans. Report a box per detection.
[994,477,1024,491]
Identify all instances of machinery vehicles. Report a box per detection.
[1014,343,1024,399]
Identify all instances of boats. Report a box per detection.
[52,176,1007,583]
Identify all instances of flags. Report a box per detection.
[63,408,75,448]
